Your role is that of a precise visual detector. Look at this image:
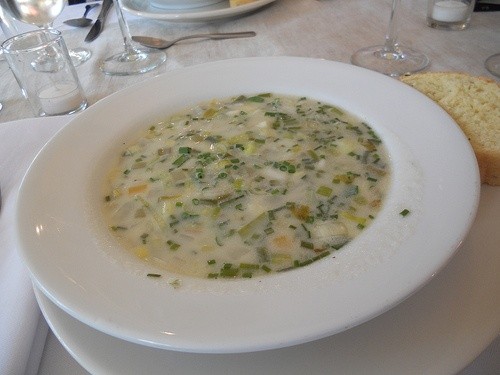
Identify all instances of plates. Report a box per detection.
[117,0,274,23]
[32,185,499,373]
[15,56,481,355]
[484,52,500,76]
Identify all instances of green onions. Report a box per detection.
[104,92,409,288]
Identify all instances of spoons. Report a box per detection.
[62,4,98,26]
[132,31,256,49]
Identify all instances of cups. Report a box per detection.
[426,0,475,31]
[0,29,87,119]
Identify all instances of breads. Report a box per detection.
[229,0,257,7]
[400,72,500,186]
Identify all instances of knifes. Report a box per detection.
[84,0,110,42]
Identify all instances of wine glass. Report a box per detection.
[100,0,167,74]
[13,0,91,73]
[352,0,430,77]
